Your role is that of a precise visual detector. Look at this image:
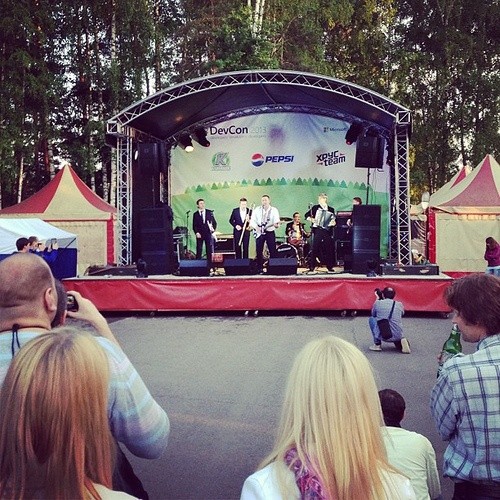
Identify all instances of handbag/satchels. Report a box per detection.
[377,319,393,340]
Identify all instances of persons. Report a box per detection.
[305,193,337,271]
[377,388,442,500]
[485,237,500,276]
[428,273,500,500]
[286,212,311,257]
[192,199,216,260]
[347,197,362,227]
[240,336,417,500]
[229,198,252,259]
[0,330,139,500]
[250,195,280,270]
[0,252,171,500]
[368,287,411,353]
[16,237,58,263]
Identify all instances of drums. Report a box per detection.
[277,242,298,259]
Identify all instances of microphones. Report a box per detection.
[210,210,214,213]
[186,210,190,214]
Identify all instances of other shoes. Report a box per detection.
[401,338,410,354]
[329,268,334,271]
[369,344,382,350]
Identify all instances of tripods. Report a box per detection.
[302,210,329,274]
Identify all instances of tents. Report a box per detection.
[427,155,500,278]
[0,218,78,281]
[0,164,117,278]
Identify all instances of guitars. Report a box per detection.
[252,220,285,239]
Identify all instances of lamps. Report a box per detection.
[178,134,194,151]
[364,125,379,140]
[190,127,210,148]
[344,121,364,146]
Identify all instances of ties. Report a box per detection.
[201,211,203,223]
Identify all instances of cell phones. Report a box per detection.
[51,238,56,248]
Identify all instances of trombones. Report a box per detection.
[238,201,255,246]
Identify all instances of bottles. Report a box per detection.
[437,323,462,378]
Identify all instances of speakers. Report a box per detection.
[333,204,381,275]
[355,137,384,168]
[136,142,167,173]
[223,259,256,275]
[139,207,174,275]
[179,260,210,276]
[267,258,298,275]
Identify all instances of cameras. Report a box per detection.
[66,296,76,309]
[374,288,384,300]
